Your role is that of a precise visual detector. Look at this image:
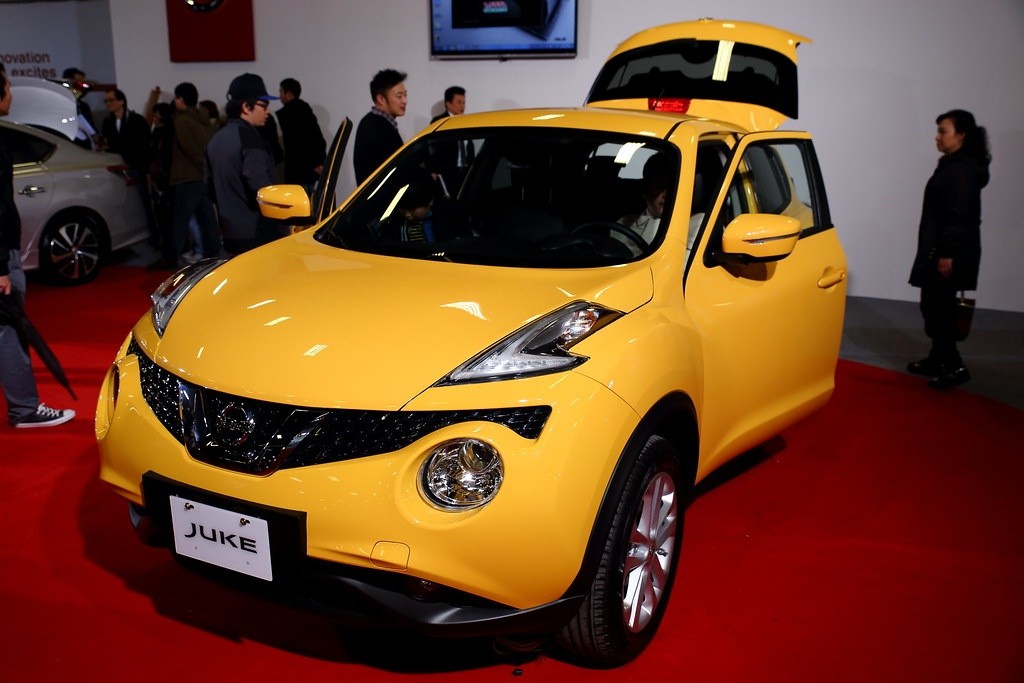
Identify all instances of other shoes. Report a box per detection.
[182,251,202,260]
[927,365,971,391]
[907,353,961,377]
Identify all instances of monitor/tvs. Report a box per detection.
[430,0,578,59]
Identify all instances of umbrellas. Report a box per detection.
[0,286,77,399]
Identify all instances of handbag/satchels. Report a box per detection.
[919,288,976,341]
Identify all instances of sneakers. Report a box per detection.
[15,403,75,427]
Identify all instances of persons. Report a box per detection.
[0,62,76,429]
[383,168,472,242]
[431,87,475,178]
[353,69,407,187]
[99,72,327,268]
[908,109,991,390]
[63,68,94,128]
[608,151,726,259]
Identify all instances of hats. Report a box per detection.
[226,73,281,101]
[388,164,438,205]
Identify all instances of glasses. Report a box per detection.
[254,102,268,111]
[103,97,116,104]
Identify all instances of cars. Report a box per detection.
[0,74,149,293]
[94,18,849,670]
